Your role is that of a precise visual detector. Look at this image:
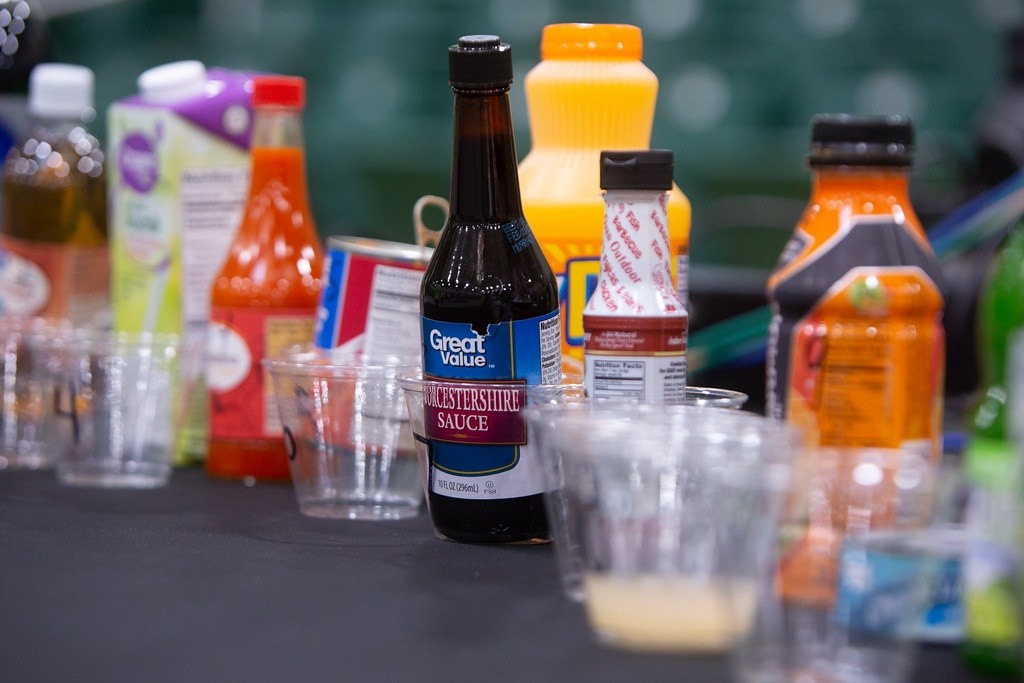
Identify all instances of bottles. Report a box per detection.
[0,63,110,425]
[511,23,696,388]
[206,73,334,479]
[420,35,565,547]
[961,209,1024,683]
[581,150,691,405]
[765,112,948,633]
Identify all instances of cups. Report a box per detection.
[564,410,806,656]
[38,333,200,488]
[530,384,751,408]
[714,446,953,683]
[519,409,762,606]
[1,318,111,472]
[258,353,423,521]
[394,367,587,545]
